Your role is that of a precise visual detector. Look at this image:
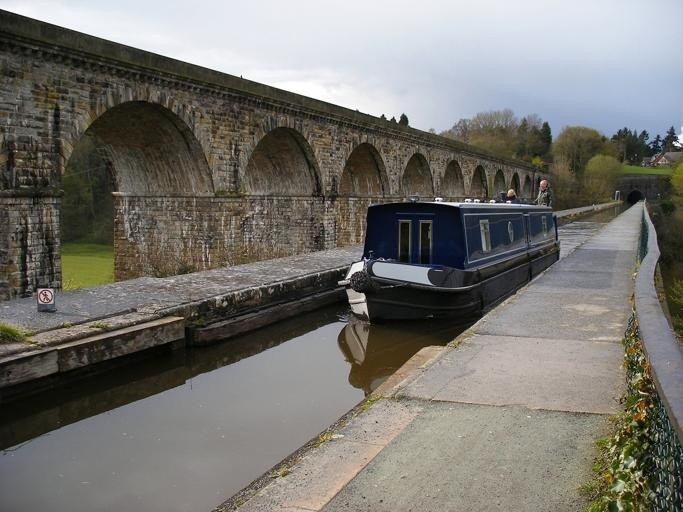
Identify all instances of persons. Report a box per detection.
[533,180,553,206]
[506,189,516,200]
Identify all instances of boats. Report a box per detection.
[336,196,560,319]
[336,320,476,395]
